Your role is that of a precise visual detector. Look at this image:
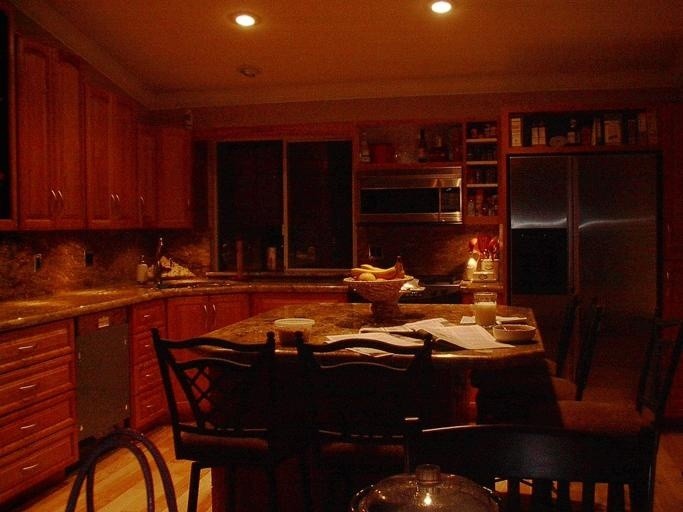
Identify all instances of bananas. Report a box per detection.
[350,256,405,279]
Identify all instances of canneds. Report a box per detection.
[603,119,623,145]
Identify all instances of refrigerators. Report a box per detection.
[508,153,663,417]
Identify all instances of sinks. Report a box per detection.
[159,276,239,288]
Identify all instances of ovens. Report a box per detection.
[73,305,131,462]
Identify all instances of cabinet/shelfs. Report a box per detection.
[461,117,507,224]
[250,293,347,318]
[138,124,157,230]
[157,123,194,230]
[86,78,138,230]
[128,294,250,434]
[355,118,466,168]
[0,318,80,507]
[498,100,683,154]
[16,36,87,230]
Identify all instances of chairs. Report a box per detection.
[149,326,313,512]
[296,329,433,512]
[527,316,683,512]
[471,295,582,386]
[421,427,659,512]
[476,304,602,423]
[65,428,178,512]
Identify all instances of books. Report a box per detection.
[323,317,517,358]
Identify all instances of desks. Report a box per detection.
[188,300,547,512]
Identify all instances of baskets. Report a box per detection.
[341,273,415,316]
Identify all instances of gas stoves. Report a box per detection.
[347,277,463,304]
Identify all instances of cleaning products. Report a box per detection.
[135,252,149,283]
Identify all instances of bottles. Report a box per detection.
[136,253,147,285]
[356,125,449,163]
[528,115,625,146]
[468,195,498,216]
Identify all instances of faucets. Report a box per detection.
[152,236,174,288]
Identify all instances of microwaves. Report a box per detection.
[354,169,463,229]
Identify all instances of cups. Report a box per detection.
[472,292,499,328]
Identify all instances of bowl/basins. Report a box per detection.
[492,324,535,342]
[272,316,316,346]
[343,274,412,299]
